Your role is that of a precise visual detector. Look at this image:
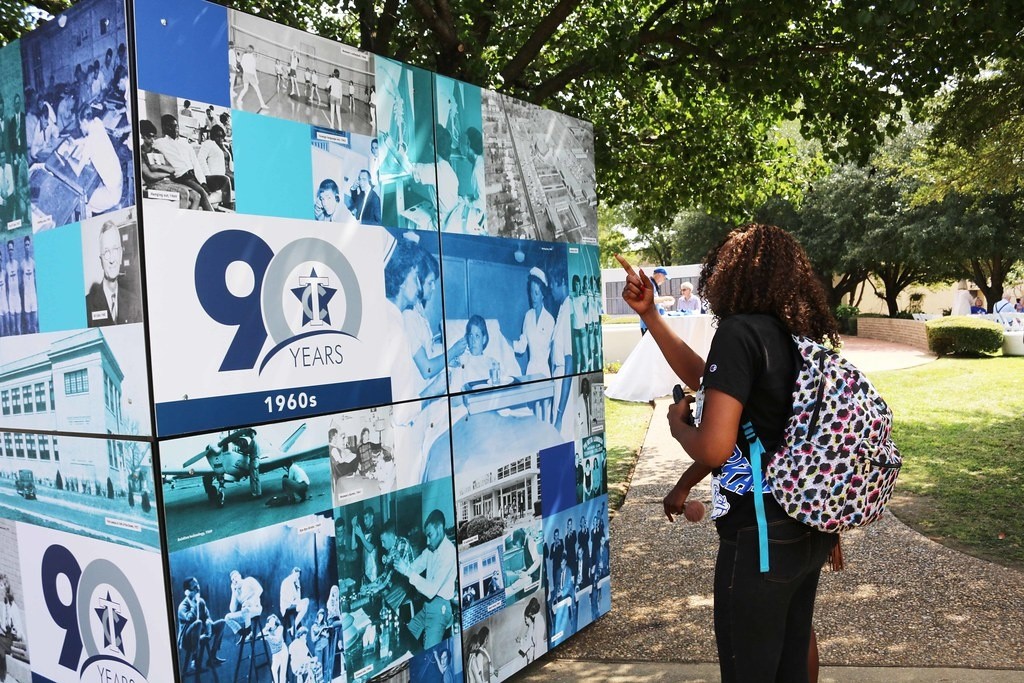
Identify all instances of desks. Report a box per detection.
[460,373,555,425]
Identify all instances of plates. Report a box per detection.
[487,376,514,386]
[472,384,491,390]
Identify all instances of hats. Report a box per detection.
[530,266,548,288]
[654,268,667,275]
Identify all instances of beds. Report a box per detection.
[422,315,562,530]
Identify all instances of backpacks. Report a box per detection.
[739,326,902,573]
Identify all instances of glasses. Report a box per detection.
[681,288,690,291]
[100,246,124,259]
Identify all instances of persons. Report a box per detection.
[676,282,702,315]
[615,220,838,683]
[1,38,611,683]
[664,454,819,683]
[950,278,1024,332]
[640,268,674,337]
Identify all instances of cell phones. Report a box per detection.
[673,384,696,426]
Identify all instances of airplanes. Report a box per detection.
[156,421,332,508]
